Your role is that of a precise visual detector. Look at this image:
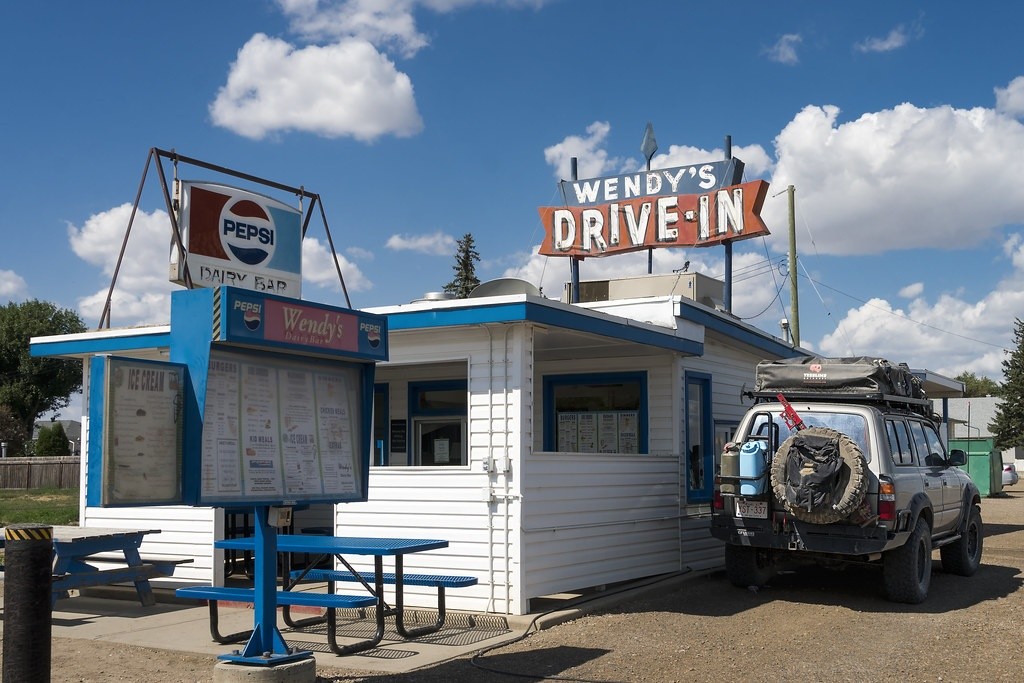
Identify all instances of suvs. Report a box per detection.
[711,355,983,605]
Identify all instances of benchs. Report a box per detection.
[78,551,194,577]
[290,569,478,627]
[176,585,379,654]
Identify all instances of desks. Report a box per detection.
[214,534,449,654]
[0,525,162,607]
